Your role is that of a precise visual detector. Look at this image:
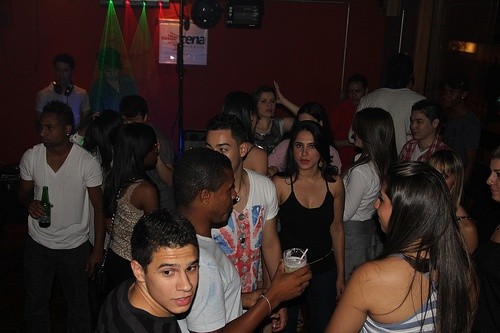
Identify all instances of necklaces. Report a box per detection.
[496,223,500,231]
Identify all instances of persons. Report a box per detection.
[172,147,313,333]
[425,147,500,333]
[101,122,161,298]
[322,159,478,333]
[205,79,346,333]
[349,53,481,164]
[326,73,370,174]
[33,53,91,130]
[69,95,175,210]
[342,106,396,287]
[96,209,200,333]
[92,47,138,115]
[16,100,106,333]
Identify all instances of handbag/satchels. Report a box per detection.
[95,265,105,293]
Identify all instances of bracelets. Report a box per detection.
[261,295,272,315]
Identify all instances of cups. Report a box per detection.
[283,248,307,272]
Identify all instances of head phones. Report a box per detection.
[54,77,74,96]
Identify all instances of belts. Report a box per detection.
[24,236,92,257]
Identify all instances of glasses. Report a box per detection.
[150,145,162,157]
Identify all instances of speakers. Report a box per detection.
[183,128,208,152]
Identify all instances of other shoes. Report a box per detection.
[296,312,305,328]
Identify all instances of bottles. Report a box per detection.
[39,186,50,228]
